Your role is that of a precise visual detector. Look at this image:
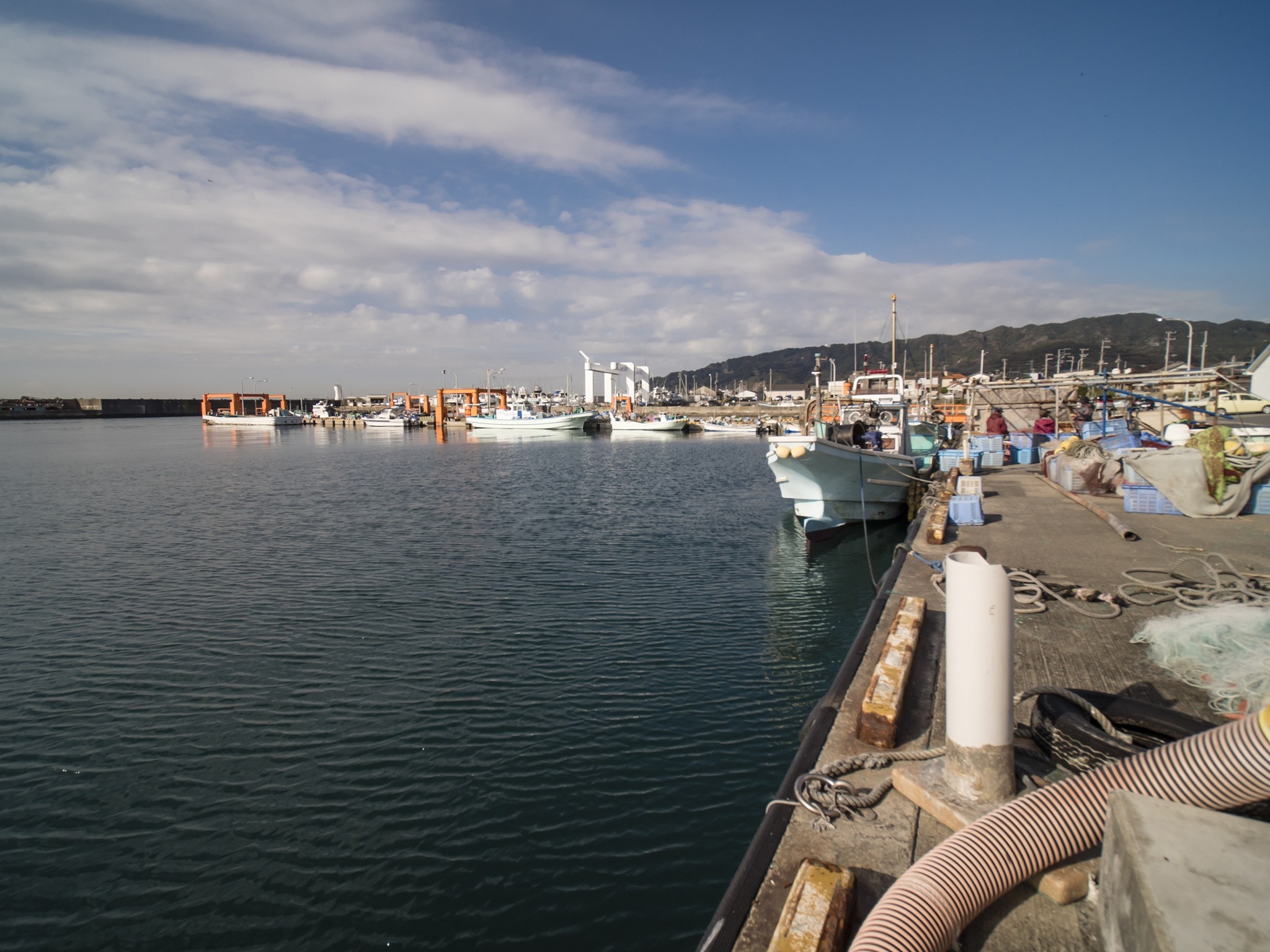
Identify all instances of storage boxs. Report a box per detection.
[938,418,1270,524]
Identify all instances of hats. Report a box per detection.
[995,407,1004,413]
[1039,409,1048,417]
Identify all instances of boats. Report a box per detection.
[607,411,688,430]
[465,401,599,429]
[203,407,304,426]
[763,353,952,530]
[700,420,770,432]
[361,408,420,427]
[445,386,585,407]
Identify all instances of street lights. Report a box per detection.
[487,368,505,415]
[442,372,459,416]
[409,383,420,413]
[1156,317,1193,402]
[289,387,292,410]
[254,380,268,408]
[241,377,254,416]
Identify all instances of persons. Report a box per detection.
[1033,410,1055,434]
[986,407,1008,435]
[859,425,883,451]
[1069,396,1095,436]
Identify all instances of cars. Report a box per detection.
[1206,393,1270,418]
